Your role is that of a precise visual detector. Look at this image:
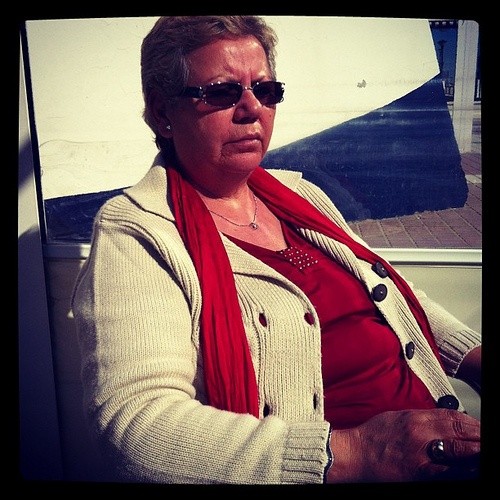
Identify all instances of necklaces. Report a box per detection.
[205,191,260,229]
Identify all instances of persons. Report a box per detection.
[81,14,483,485]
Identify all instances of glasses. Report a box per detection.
[148,78,291,109]
